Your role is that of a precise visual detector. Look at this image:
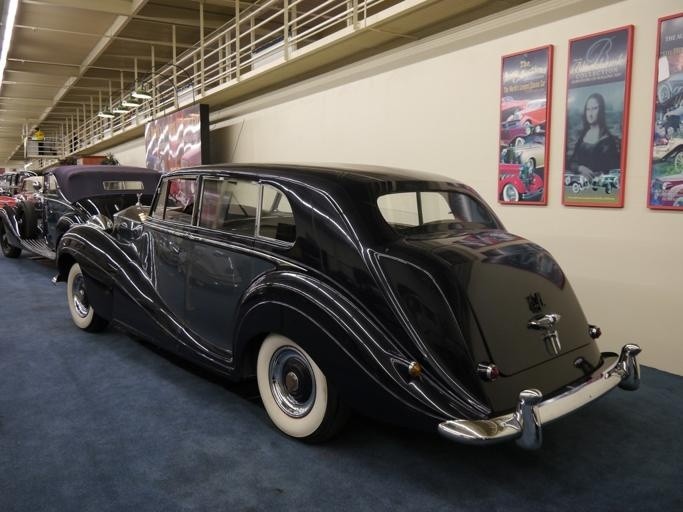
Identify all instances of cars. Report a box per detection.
[0,164,163,260]
[50,163,643,452]
[502,96,546,147]
[652,138,683,208]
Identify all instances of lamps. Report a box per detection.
[94,62,197,120]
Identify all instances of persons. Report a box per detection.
[69,132,81,152]
[567,93,620,181]
[32,127,45,155]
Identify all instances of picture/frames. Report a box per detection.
[496,14,682,213]
